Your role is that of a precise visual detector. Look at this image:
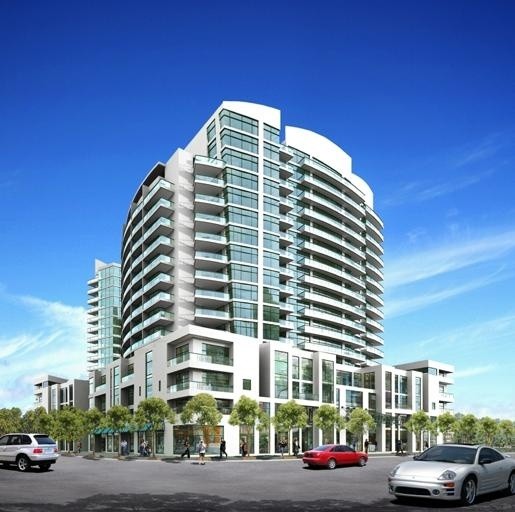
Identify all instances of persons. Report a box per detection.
[179,434,302,466]
[74,437,151,457]
[364,439,371,454]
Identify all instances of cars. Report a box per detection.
[386,444,515,506]
[303,444,369,470]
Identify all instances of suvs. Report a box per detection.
[0,433,59,472]
